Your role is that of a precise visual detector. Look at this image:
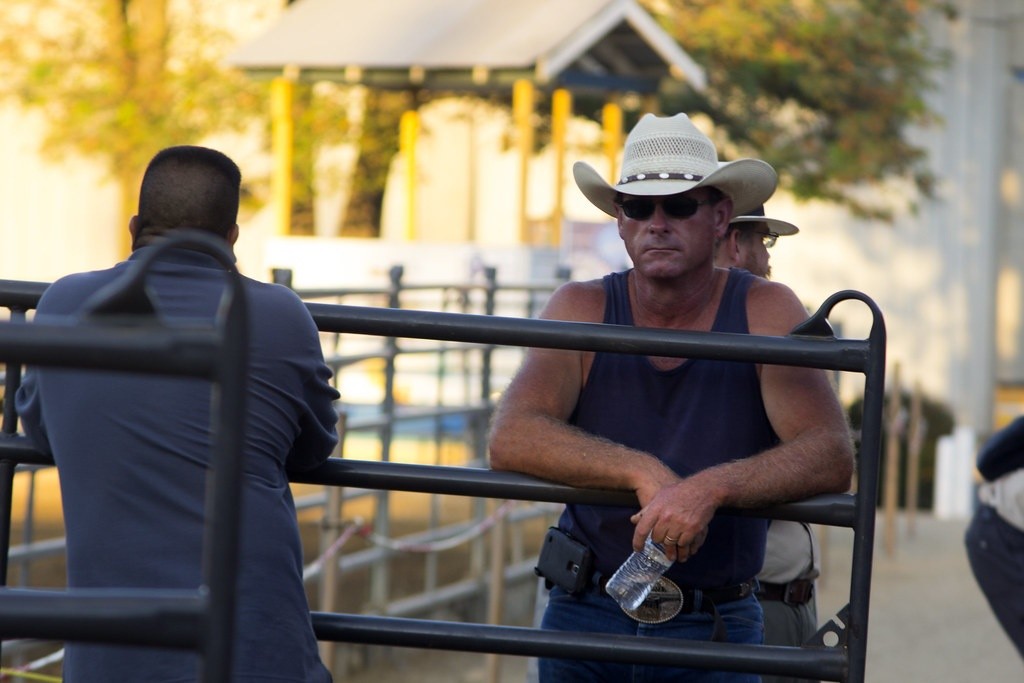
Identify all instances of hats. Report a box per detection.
[728,204,800,236]
[571,112,781,219]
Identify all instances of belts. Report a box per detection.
[763,576,813,608]
[600,579,757,642]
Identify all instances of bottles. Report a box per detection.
[606,533,677,612]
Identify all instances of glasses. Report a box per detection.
[617,191,715,221]
[743,230,779,248]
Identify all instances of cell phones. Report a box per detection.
[535,528,588,594]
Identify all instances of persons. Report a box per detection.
[15,143,340,683]
[485,112,856,683]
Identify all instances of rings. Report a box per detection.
[665,535,678,542]
